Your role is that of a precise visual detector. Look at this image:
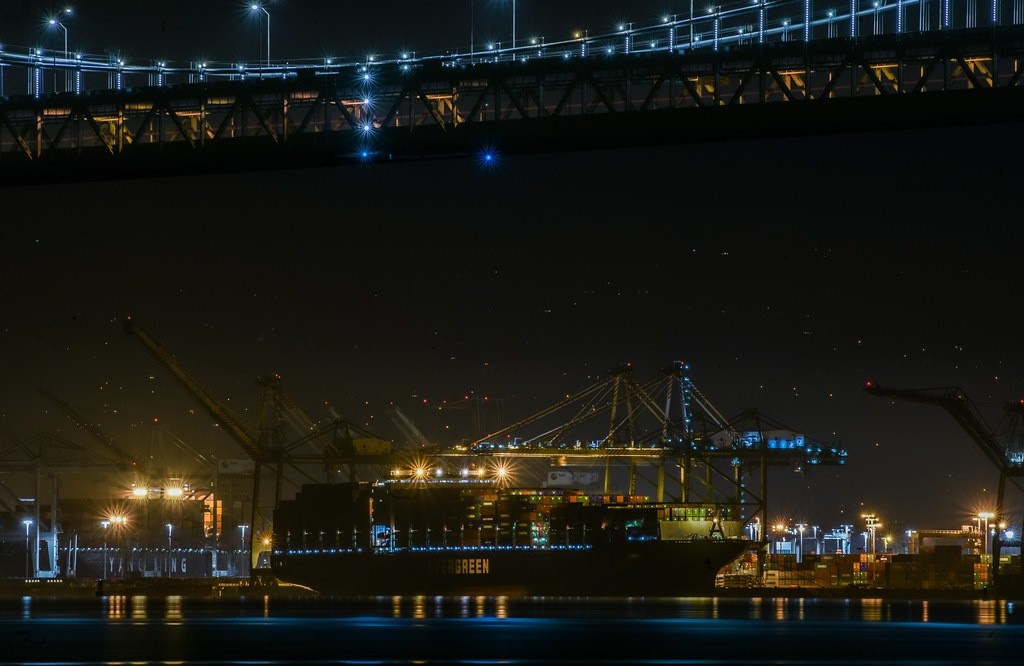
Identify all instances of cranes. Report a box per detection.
[0,309,850,541]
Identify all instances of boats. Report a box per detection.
[210,566,322,601]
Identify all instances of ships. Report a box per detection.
[267,478,770,597]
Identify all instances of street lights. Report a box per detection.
[166,523,173,578]
[861,514,882,589]
[109,511,129,579]
[972,511,995,554]
